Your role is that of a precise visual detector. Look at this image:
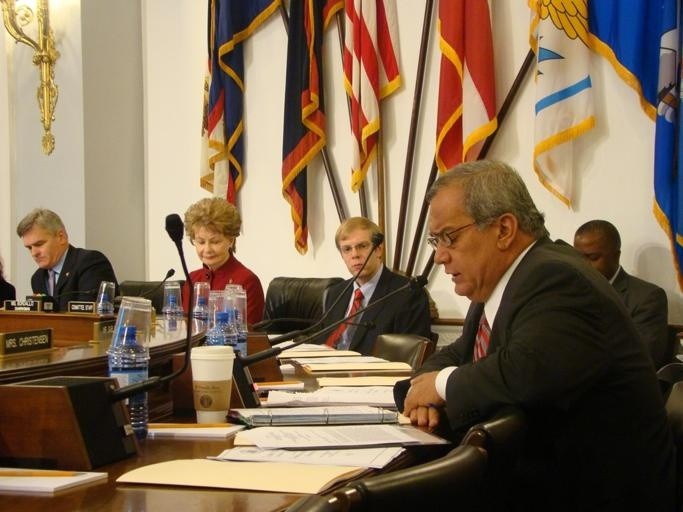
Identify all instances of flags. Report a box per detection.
[432,2,500,174]
[190,0,287,207]
[332,0,405,196]
[525,0,680,307]
[276,1,348,255]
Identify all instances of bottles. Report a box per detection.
[96,293,114,313]
[107,326,149,445]
[162,295,183,339]
[207,309,248,357]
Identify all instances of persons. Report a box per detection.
[173,197,266,325]
[318,212,434,367]
[16,206,121,315]
[387,153,673,511]
[572,216,670,391]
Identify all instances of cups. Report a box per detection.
[193,281,249,334]
[189,345,236,424]
[104,295,152,360]
[161,281,184,312]
[96,281,116,312]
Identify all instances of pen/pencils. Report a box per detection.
[146,422,235,428]
[258,381,301,385]
[0,470,83,477]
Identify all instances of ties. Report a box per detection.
[327,288,364,346]
[472,311,493,362]
[48,268,56,297]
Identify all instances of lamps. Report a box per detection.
[0,0,60,158]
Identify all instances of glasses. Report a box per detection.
[426,210,509,251]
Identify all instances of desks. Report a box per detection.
[0,302,415,512]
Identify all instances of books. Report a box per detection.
[0,464,108,492]
[113,332,453,493]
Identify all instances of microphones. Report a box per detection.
[248,232,384,381]
[52,289,98,299]
[172,275,428,419]
[115,268,175,312]
[0,213,193,470]
